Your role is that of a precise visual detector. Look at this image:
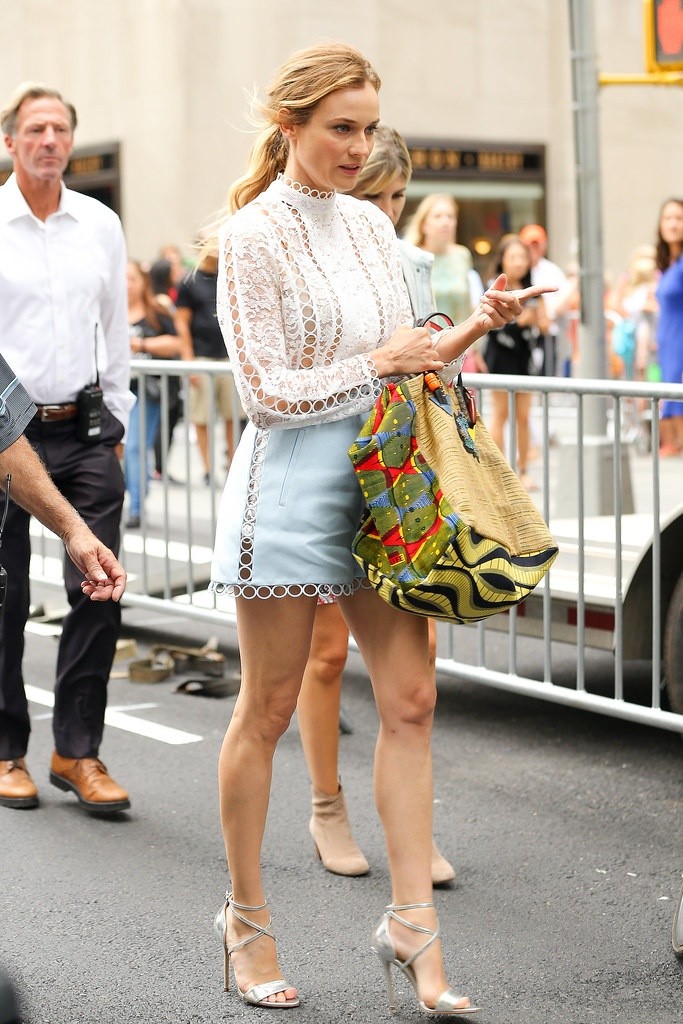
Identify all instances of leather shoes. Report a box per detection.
[1,758,39,808]
[50,749,129,811]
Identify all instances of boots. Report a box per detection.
[308,784,370,875]
[432,838,456,883]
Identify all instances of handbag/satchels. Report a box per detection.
[351,312,558,626]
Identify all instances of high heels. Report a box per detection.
[375,902,482,1014]
[214,890,300,1008]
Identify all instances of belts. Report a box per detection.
[30,405,76,421]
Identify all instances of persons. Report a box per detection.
[310,127,459,888]
[176,224,243,491]
[516,223,573,391]
[121,256,187,530]
[652,197,683,455]
[554,238,670,453]
[210,41,560,1024]
[151,242,214,491]
[1,353,132,1024]
[479,232,552,481]
[403,191,485,387]
[1,80,140,820]
